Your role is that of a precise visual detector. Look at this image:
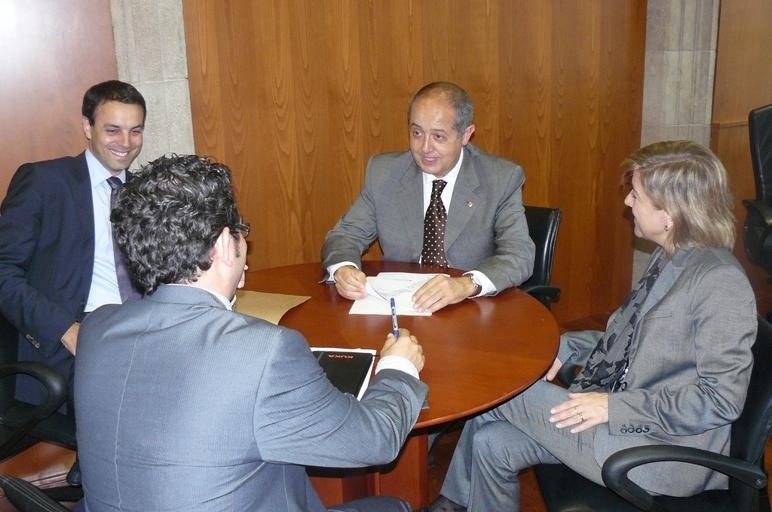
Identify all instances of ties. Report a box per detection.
[422,180,447,267]
[106,176,143,301]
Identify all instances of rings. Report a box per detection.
[579,412,584,422]
[574,405,578,414]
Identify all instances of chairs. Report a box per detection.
[743,103,772,322]
[517,204,562,310]
[534,313,772,512]
[0,313,85,512]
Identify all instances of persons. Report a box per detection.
[320,83,536,469]
[423,140,759,512]
[0,80,146,418]
[73,155,426,512]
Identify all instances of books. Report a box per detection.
[348,271,451,317]
[310,351,373,399]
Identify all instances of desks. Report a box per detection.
[235,261,560,511]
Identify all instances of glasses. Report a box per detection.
[233,221,250,237]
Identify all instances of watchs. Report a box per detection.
[465,273,482,297]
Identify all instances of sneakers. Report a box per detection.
[417,495,467,512]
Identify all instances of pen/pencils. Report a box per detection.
[391,297,400,338]
